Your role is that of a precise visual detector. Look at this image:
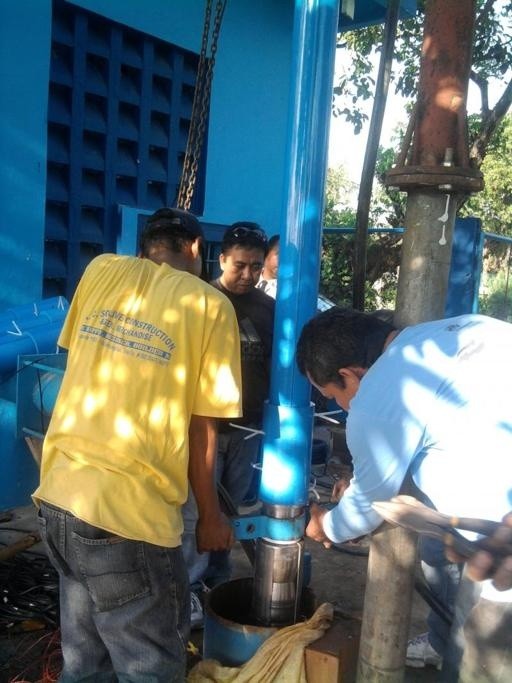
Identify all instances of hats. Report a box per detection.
[148,207,209,255]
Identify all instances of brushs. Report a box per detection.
[0,620,47,635]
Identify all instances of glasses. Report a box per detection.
[225,226,271,245]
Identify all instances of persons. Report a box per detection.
[30,209,234,683]
[442,509,510,591]
[256,236,279,299]
[181,222,276,627]
[293,308,510,682]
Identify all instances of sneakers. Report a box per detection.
[402,628,449,670]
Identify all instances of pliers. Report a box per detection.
[371,500,512,570]
